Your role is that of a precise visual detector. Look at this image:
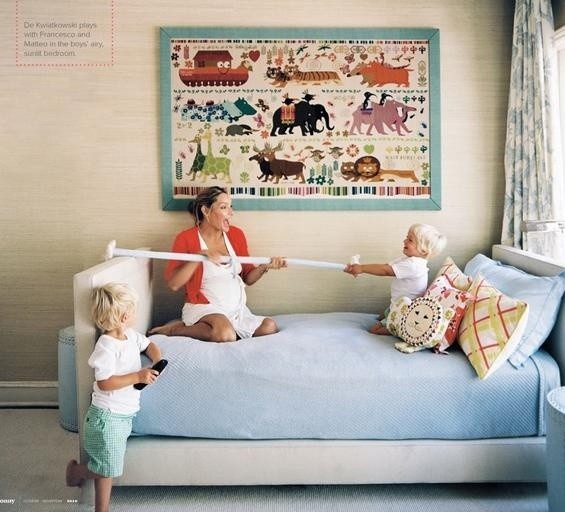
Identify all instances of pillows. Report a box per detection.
[386,253,565,380]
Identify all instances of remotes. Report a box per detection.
[133,359,168,390]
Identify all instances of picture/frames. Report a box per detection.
[160,27,441,211]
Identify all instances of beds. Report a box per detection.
[73,245,565,504]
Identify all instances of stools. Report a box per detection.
[57,326,79,432]
[546,387,565,512]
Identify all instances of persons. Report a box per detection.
[64,281,163,511]
[143,186,288,342]
[343,223,450,335]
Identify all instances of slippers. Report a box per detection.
[66,459,81,487]
